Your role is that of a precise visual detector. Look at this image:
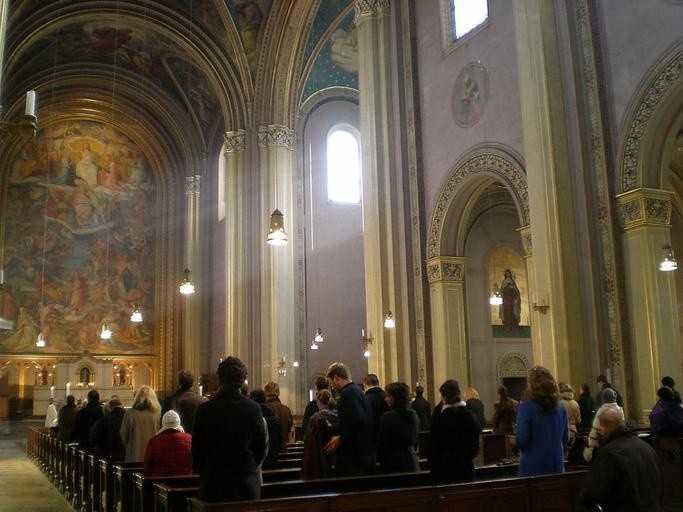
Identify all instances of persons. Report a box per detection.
[500,269,521,332]
[492,366,683,512]
[46,357,293,504]
[302,363,486,485]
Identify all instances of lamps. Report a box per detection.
[479,1,504,305]
[657,224,678,271]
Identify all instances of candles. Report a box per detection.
[50,386,55,397]
[65,383,71,397]
[25,88,36,115]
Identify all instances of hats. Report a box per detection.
[560,384,575,392]
[602,388,617,403]
[162,410,181,429]
